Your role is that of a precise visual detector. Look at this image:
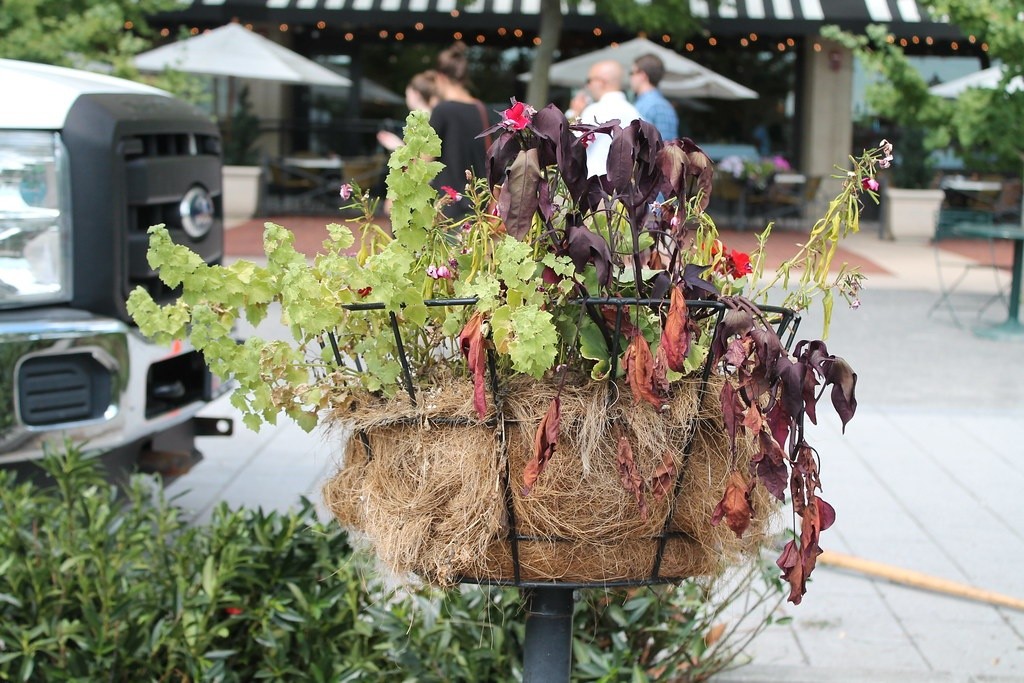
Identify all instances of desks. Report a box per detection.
[952,222,1024,335]
[285,151,346,212]
[943,176,1004,212]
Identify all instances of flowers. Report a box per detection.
[718,154,790,191]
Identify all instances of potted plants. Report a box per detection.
[881,112,946,246]
[215,84,265,230]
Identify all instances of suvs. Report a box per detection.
[0,53,236,524]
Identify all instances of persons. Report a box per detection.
[565,62,644,224]
[629,53,678,267]
[377,48,490,251]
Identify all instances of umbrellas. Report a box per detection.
[518,36,759,99]
[312,63,400,102]
[929,63,1024,100]
[117,24,350,114]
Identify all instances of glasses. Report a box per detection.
[586,77,603,85]
[630,69,643,76]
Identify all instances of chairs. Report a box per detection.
[717,172,775,230]
[775,176,824,233]
[925,201,1016,333]
